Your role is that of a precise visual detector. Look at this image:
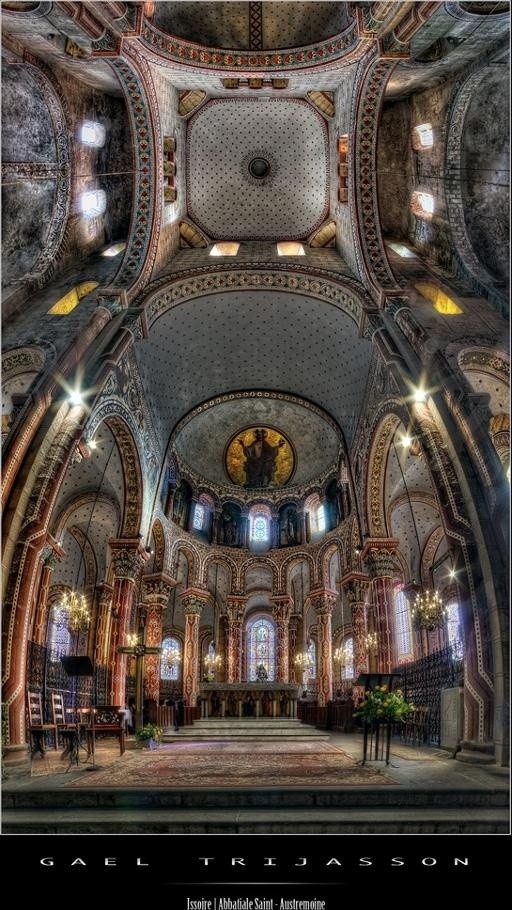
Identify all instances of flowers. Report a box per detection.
[136,723,164,740]
[351,683,417,725]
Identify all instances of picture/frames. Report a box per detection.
[221,423,298,487]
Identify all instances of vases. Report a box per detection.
[144,738,158,749]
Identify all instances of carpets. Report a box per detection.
[30,751,93,777]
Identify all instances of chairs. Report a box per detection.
[392,705,431,746]
[51,693,90,755]
[84,703,127,756]
[26,690,58,752]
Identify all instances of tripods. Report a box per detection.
[61,676,89,766]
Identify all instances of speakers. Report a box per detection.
[63,656,93,676]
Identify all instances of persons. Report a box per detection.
[238,429,285,488]
[256,664,268,683]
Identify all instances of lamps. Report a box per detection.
[55,439,448,676]
